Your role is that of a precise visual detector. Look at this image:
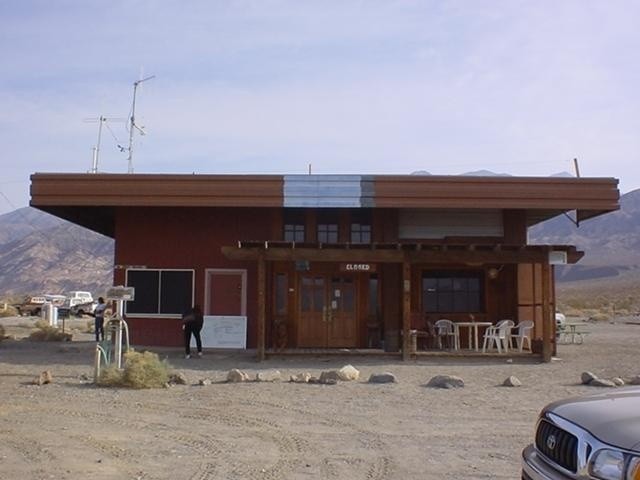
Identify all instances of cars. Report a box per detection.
[515,382,639,479]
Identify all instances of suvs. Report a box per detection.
[12,288,99,318]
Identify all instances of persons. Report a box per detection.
[181,308,203,359]
[94,297,106,341]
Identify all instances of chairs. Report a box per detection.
[405,310,591,355]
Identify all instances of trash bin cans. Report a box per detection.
[385,330,399,352]
